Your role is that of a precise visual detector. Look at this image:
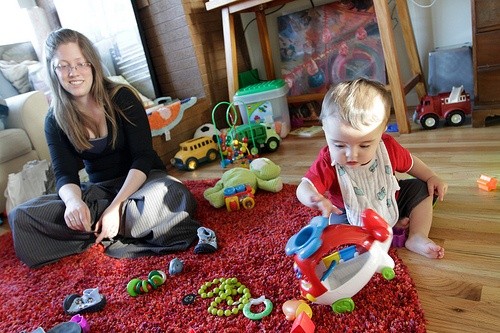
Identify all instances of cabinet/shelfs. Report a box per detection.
[471,0,500,109]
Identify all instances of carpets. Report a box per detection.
[0,179,428,333]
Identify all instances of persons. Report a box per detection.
[295,76,449,259]
[7,28,198,268]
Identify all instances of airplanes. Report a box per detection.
[284,209,394,314]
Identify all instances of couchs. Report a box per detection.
[0,53,50,212]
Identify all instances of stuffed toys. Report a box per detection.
[203,158,283,209]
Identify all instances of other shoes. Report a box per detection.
[473,114,500,127]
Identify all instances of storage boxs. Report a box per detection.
[428,42,473,96]
[233,77,292,140]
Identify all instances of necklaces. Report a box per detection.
[198,277,251,316]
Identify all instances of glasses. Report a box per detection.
[54,62,91,74]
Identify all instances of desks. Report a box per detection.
[204,0,428,134]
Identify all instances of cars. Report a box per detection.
[174,136,219,170]
[223,122,282,158]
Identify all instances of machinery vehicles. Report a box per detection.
[413,85,471,130]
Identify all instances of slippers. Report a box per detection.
[20,322,82,333]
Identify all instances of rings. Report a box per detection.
[70,219,75,221]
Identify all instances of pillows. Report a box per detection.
[106,70,155,108]
